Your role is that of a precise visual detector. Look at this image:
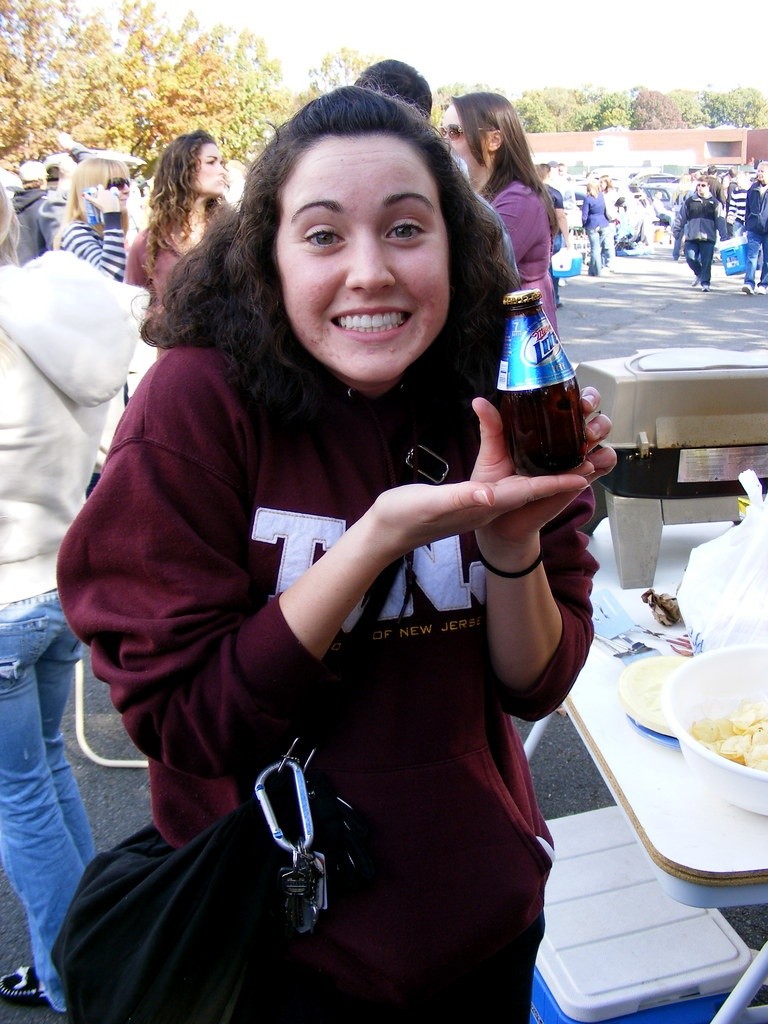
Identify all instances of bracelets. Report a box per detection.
[477,548,543,579]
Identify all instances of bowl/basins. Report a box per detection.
[662,640,768,818]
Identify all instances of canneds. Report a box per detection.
[81,186,102,225]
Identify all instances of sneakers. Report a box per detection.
[742,285,755,295]
[758,285,766,295]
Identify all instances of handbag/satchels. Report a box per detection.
[50,759,351,1024]
[677,468,767,654]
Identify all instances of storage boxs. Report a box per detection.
[721,241,760,273]
[550,256,582,278]
[528,805,752,1024]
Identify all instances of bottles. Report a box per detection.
[494,287,587,477]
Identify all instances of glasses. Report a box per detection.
[438,124,496,140]
[107,177,130,191]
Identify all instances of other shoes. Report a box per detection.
[0,967,48,1004]
[692,274,701,287]
[702,284,709,291]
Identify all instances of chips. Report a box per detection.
[691,702,768,773]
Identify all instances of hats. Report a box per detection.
[20,160,50,181]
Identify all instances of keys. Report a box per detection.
[276,868,312,929]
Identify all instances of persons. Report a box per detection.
[581,175,620,276]
[351,57,468,181]
[535,160,576,310]
[654,190,673,226]
[0,183,153,1011]
[439,91,559,342]
[123,128,238,364]
[0,130,247,284]
[56,88,617,1023]
[671,157,768,295]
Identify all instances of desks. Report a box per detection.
[516,510,767,1024]
